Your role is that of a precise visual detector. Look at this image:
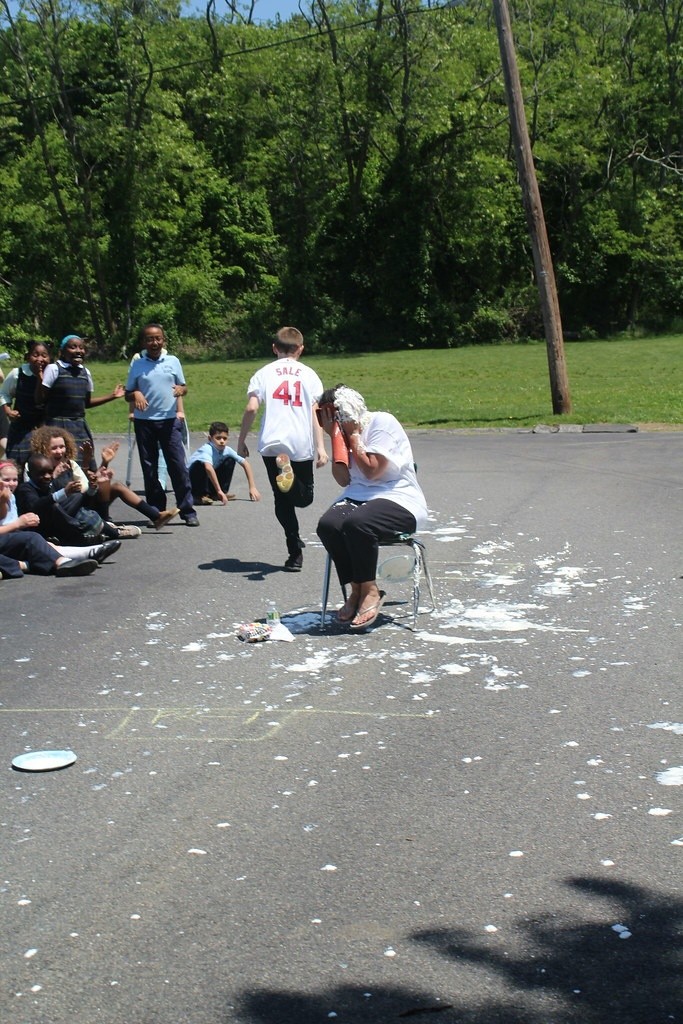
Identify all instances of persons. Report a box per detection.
[42,335,127,471]
[0,461,122,581]
[189,422,260,506]
[0,339,54,484]
[238,326,329,574]
[317,382,428,629]
[124,323,199,527]
[13,454,106,547]
[23,426,142,538]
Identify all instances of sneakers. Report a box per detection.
[88,540,121,564]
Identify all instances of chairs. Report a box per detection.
[319,462,437,632]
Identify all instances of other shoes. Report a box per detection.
[194,496,213,505]
[225,494,236,500]
[81,533,105,546]
[27,560,54,575]
[116,524,141,538]
[186,517,199,526]
[276,454,294,493]
[147,519,155,528]
[284,549,303,571]
[105,521,116,529]
[153,508,180,530]
[56,559,98,576]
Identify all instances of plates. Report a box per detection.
[11,750,78,771]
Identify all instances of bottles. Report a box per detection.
[266,601,281,639]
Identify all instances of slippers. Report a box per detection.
[336,605,360,624]
[350,590,386,628]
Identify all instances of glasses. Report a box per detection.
[144,337,165,344]
